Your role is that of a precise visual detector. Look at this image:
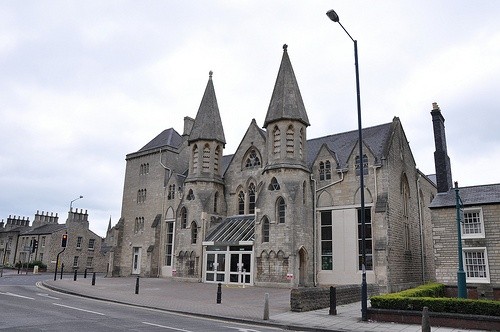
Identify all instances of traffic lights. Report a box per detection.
[31,239,38,254]
[61,234,68,248]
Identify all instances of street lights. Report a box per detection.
[326,8,370,322]
